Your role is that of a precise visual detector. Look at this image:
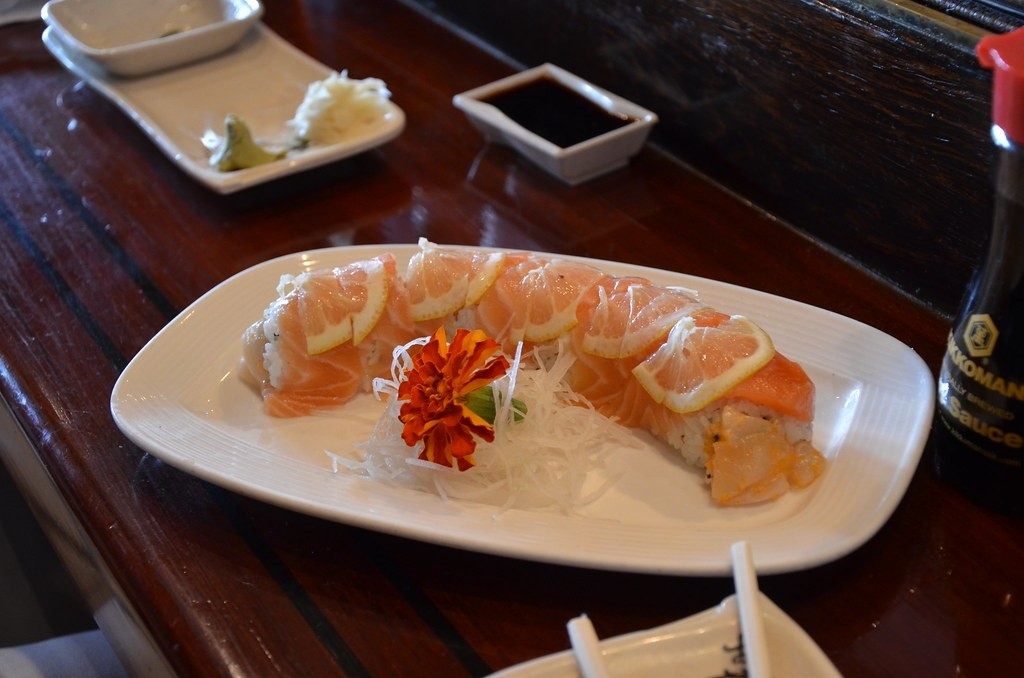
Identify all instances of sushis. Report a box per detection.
[241,243,828,506]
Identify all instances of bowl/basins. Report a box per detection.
[40,0,265,80]
[452,61,660,186]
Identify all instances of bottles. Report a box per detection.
[936,25,1024,488]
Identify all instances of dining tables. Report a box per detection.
[0,1,1023,678]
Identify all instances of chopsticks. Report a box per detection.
[566,540,774,678]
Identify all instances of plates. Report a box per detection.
[0,0,51,26]
[485,589,845,678]
[41,19,406,196]
[111,244,936,577]
